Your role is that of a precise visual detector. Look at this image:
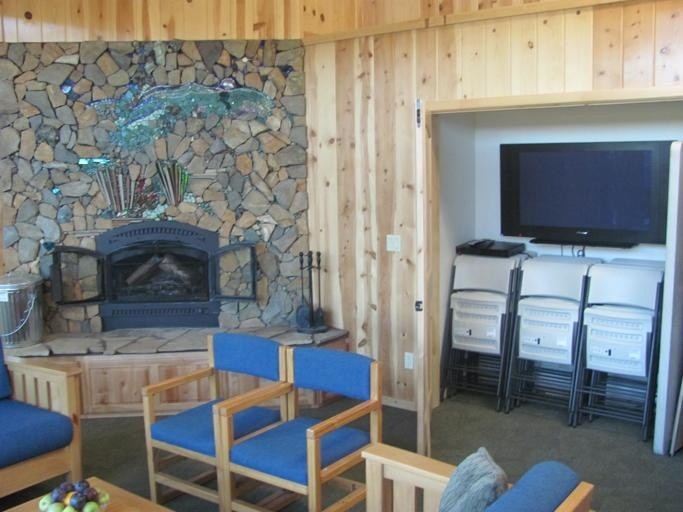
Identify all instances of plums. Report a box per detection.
[51,480,97,510]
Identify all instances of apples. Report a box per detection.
[39,488,111,512]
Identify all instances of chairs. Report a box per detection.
[1,344,88,505]
[141,332,382,512]
[360,441,593,512]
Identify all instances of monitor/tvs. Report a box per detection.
[499,139,674,249]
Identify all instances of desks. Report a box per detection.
[2,477,182,512]
